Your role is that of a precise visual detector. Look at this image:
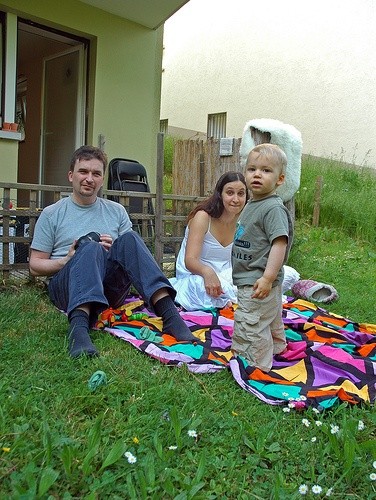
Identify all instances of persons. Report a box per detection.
[231,142,295,376]
[28,144,202,356]
[168,170,250,310]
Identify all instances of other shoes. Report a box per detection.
[292,280,339,303]
